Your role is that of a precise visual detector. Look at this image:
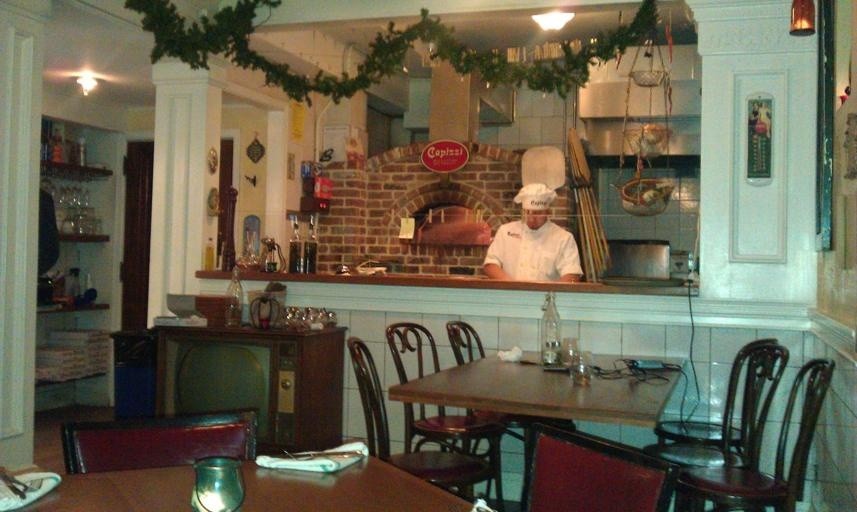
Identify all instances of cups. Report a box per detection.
[570,349,592,388]
[563,338,578,367]
[61,215,102,235]
[189,458,246,512]
[40,144,54,161]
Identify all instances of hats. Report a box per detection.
[513,183,557,211]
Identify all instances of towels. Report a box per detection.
[255,441,371,472]
[0,471,63,512]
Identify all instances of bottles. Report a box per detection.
[204,238,215,271]
[541,290,560,364]
[52,127,65,163]
[288,223,304,273]
[78,144,86,168]
[304,223,317,274]
[224,267,243,330]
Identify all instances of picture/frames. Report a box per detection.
[817,0,832,253]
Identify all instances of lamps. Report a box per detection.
[532,8,576,32]
[76,74,98,96]
[788,1,816,35]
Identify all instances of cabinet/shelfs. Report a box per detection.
[155,316,347,453]
[36,159,114,387]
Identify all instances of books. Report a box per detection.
[35,329,109,383]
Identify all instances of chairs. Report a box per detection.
[655,337,779,445]
[347,337,495,510]
[521,424,680,512]
[385,322,503,498]
[674,357,835,512]
[61,408,257,473]
[643,344,789,512]
[447,322,529,510]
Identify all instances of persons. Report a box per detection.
[748,101,771,138]
[483,183,584,282]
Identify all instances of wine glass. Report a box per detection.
[40,177,92,209]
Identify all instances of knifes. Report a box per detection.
[269,451,359,457]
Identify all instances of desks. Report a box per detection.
[109,331,156,418]
